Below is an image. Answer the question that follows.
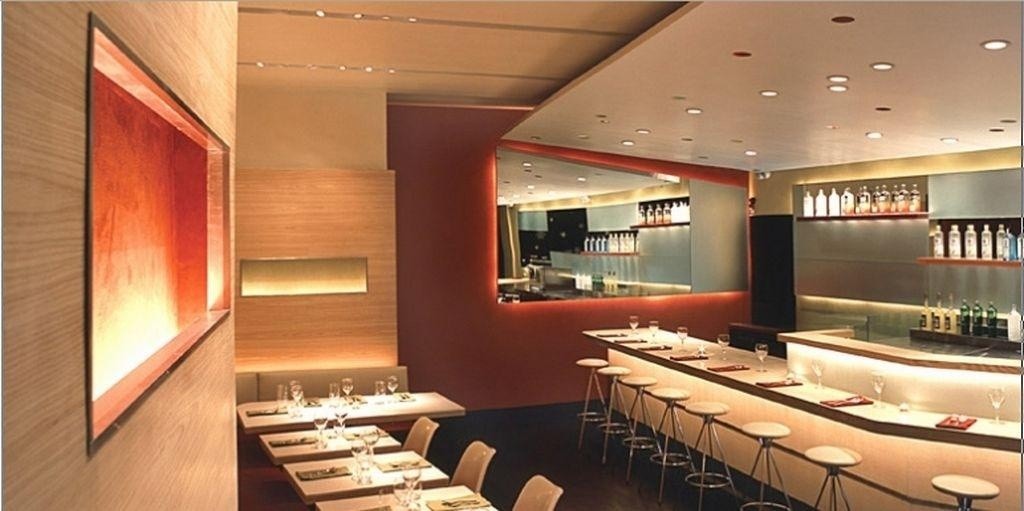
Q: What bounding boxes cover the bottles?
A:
[576,270,618,295]
[803,191,814,217]
[583,231,635,253]
[960,299,970,334]
[1008,304,1022,343]
[664,203,671,224]
[854,183,922,213]
[816,189,827,216]
[933,225,944,258]
[996,225,1005,260]
[828,187,840,216]
[647,204,655,225]
[920,295,932,331]
[671,202,690,222]
[656,204,663,224]
[964,225,977,260]
[944,294,957,334]
[932,292,944,333]
[981,224,993,259]
[987,301,997,337]
[972,301,982,335]
[840,188,855,215]
[639,206,646,225]
[948,225,961,258]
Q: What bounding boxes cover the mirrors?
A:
[494,146,751,306]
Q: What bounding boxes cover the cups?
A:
[360,426,380,466]
[342,377,354,397]
[649,321,659,344]
[334,405,349,435]
[402,461,422,492]
[411,482,422,508]
[717,334,730,361]
[628,315,639,335]
[359,466,372,485]
[811,358,825,392]
[313,411,328,441]
[290,385,304,408]
[986,384,1008,428]
[755,343,768,373]
[277,383,288,408]
[387,374,399,396]
[870,369,886,410]
[328,383,340,402]
[350,440,368,475]
[677,326,687,347]
[394,478,407,506]
[374,381,386,398]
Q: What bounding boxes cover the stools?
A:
[801,445,864,511]
[574,358,617,448]
[930,474,1000,511]
[649,386,698,501]
[595,365,638,466]
[619,375,664,483]
[682,401,737,511]
[737,420,794,511]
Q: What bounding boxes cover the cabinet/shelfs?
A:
[909,218,1023,352]
[796,213,930,223]
[776,327,1024,424]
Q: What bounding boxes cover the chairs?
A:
[402,414,443,460]
[509,474,564,511]
[451,439,499,496]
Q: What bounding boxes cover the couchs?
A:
[235,366,409,436]
